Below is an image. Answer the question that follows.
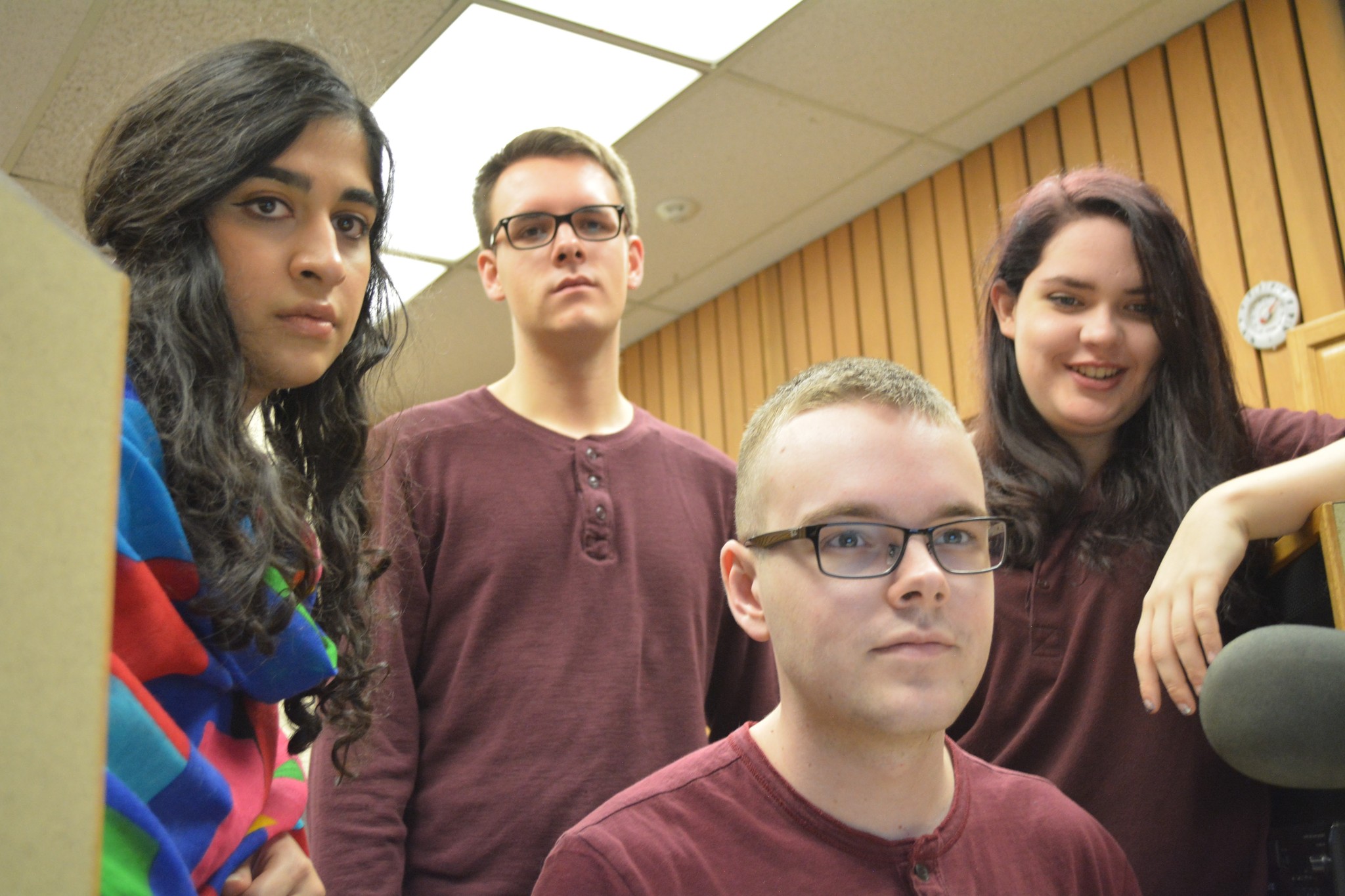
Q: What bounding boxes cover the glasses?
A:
[490,204,628,250]
[744,515,1010,579]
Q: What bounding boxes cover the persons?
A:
[312,127,786,896]
[79,36,409,896]
[963,169,1344,896]
[530,354,1144,896]
